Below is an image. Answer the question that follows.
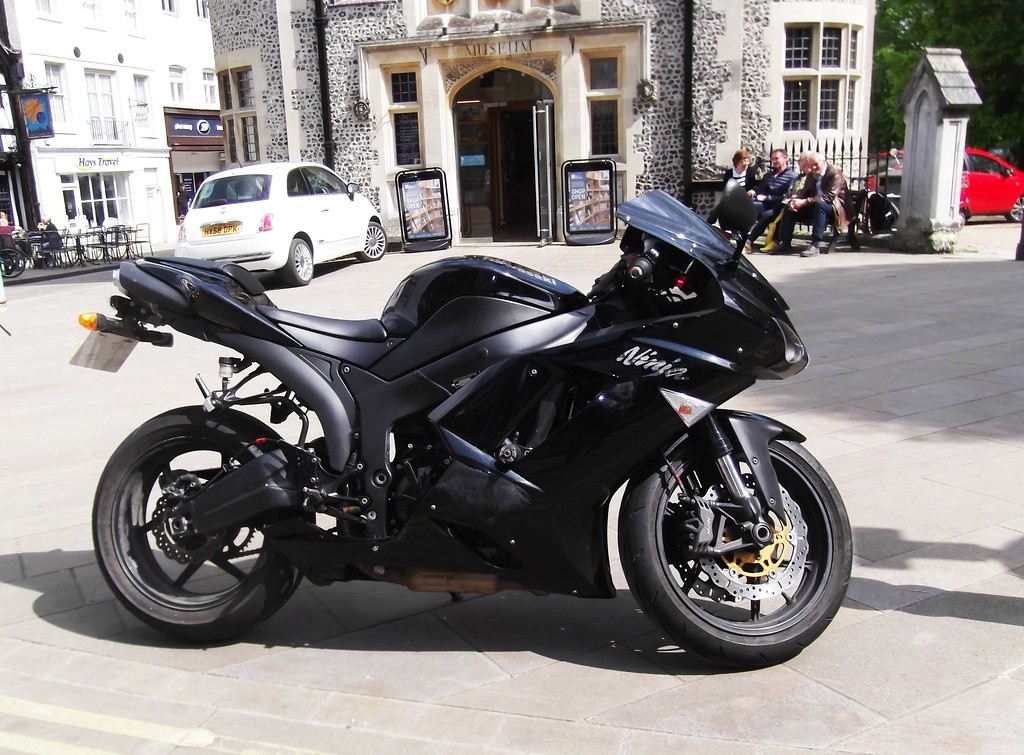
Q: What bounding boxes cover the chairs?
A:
[0,223,153,275]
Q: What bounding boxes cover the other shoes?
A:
[760,242,777,252]
[767,240,792,254]
[801,245,821,256]
[744,244,753,254]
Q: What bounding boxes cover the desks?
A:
[15,237,42,266]
[63,233,102,268]
[110,229,143,262]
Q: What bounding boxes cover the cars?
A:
[177,163,387,284]
[884,150,1024,227]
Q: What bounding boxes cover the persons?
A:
[769,152,858,258]
[36,215,64,269]
[722,149,811,254]
[0,209,10,226]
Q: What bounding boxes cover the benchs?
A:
[826,191,868,255]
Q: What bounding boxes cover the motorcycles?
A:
[74,189,854,672]
